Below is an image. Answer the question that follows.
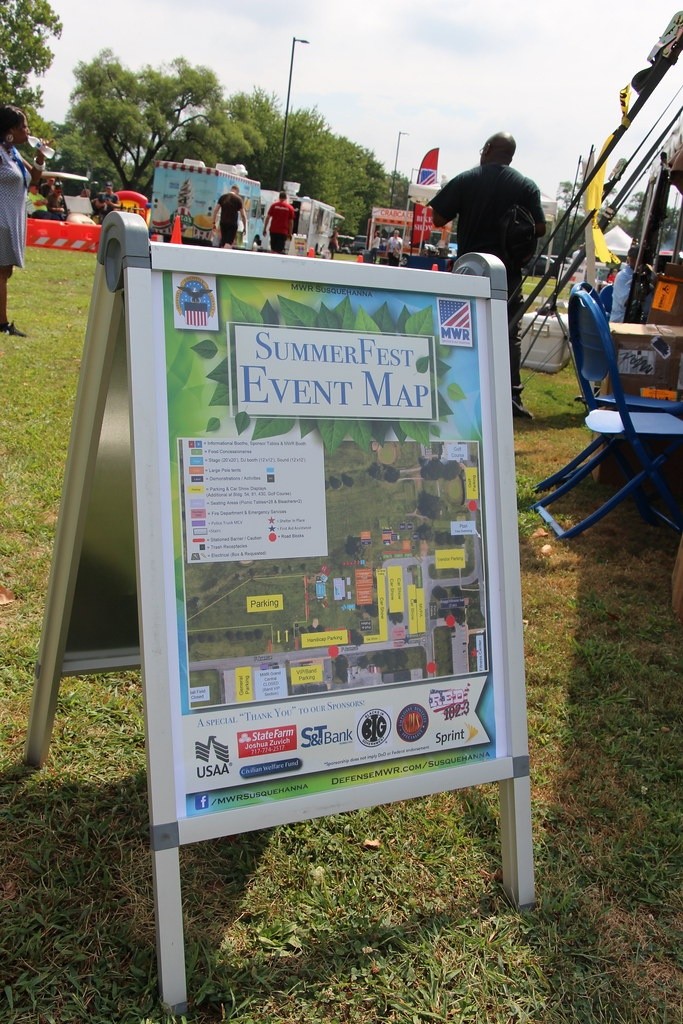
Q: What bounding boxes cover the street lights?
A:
[390,132,409,208]
[277,37,310,189]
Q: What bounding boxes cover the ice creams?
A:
[177,177,193,212]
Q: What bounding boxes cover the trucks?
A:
[265,190,345,257]
[148,158,264,252]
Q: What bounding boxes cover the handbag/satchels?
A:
[393,247,400,259]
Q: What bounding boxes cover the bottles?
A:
[27,135,55,159]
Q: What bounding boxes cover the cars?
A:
[521,255,584,279]
[398,243,455,272]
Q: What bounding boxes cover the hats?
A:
[106,181,112,188]
[628,247,639,259]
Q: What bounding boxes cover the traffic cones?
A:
[170,215,183,243]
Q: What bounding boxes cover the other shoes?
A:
[0,321,26,338]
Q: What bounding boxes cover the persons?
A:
[426,132,548,420]
[608,247,639,323]
[385,230,403,266]
[74,174,122,225]
[262,190,296,254]
[26,176,70,221]
[366,231,381,264]
[328,227,340,259]
[211,184,247,249]
[0,104,50,338]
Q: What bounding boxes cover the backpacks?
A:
[497,202,538,268]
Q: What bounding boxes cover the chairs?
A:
[527,281,683,537]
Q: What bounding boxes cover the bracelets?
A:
[34,156,46,171]
[212,222,215,224]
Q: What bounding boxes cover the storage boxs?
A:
[588,320,683,486]
[520,310,571,374]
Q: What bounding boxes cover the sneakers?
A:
[511,384,535,420]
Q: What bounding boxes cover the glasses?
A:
[479,146,485,156]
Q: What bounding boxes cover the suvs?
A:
[351,235,366,254]
